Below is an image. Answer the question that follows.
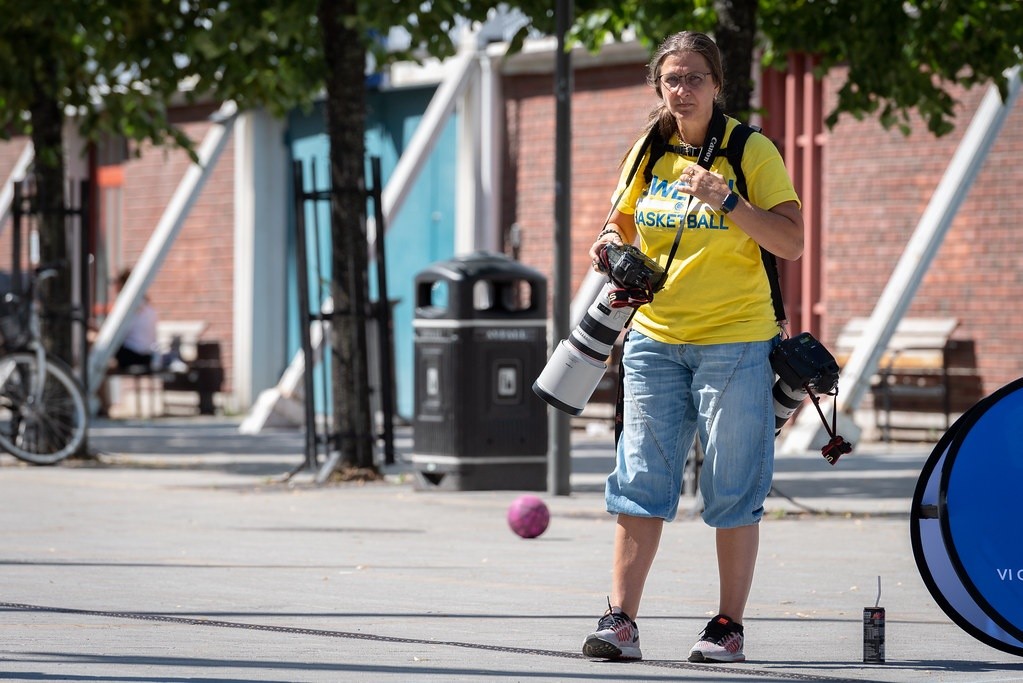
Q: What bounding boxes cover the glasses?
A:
[658,72,711,89]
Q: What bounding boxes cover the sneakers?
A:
[687,615,746,662]
[582,595,642,659]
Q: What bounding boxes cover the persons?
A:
[86,269,156,420]
[583,33,804,664]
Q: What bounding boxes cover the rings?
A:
[689,170,694,176]
[689,177,692,183]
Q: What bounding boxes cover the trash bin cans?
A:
[402,249,550,489]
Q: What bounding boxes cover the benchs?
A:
[117,317,212,417]
[824,316,961,447]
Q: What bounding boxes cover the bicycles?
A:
[0,292,88,466]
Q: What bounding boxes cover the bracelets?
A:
[597,229,623,243]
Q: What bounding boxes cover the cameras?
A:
[531,243,670,418]
[770,331,841,438]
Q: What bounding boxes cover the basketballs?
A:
[508,496,549,539]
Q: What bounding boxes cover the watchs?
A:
[712,191,739,216]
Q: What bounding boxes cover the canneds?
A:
[862,606,886,664]
